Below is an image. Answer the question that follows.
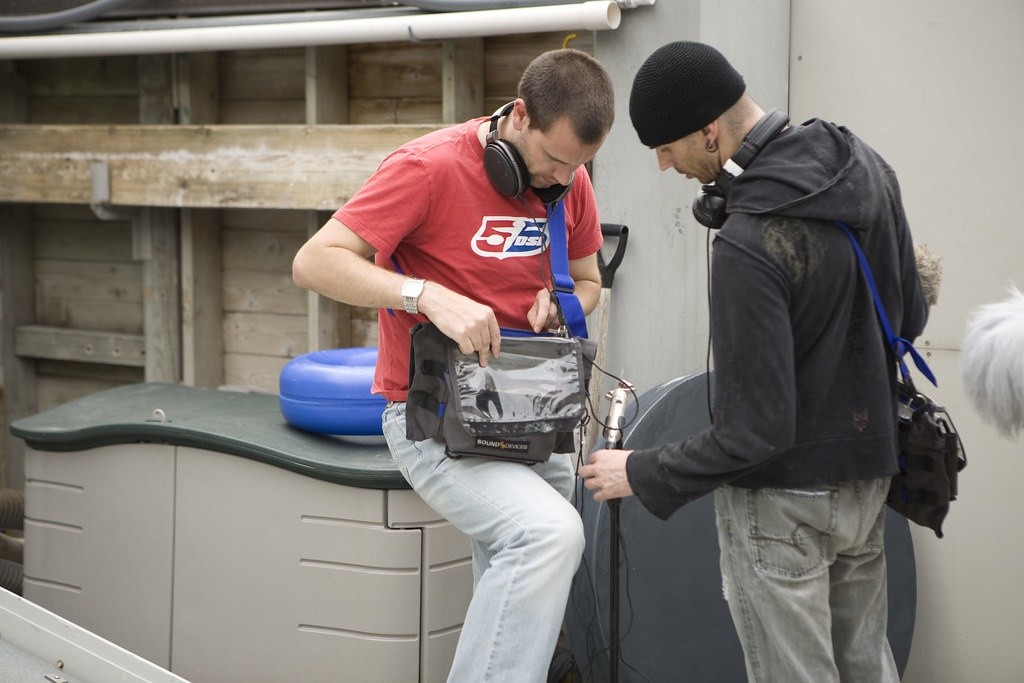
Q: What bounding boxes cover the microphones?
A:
[607,389,625,451]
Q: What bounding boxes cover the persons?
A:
[293,50,614,683]
[581,42,926,683]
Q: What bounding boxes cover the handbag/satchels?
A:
[406,322,598,464]
[885,383,966,538]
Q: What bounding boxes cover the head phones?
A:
[484,101,574,205]
[692,107,789,229]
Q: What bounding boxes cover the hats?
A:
[629,41,746,150]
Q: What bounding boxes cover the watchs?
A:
[402,276,426,314]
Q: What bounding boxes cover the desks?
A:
[6,381,474,683]
